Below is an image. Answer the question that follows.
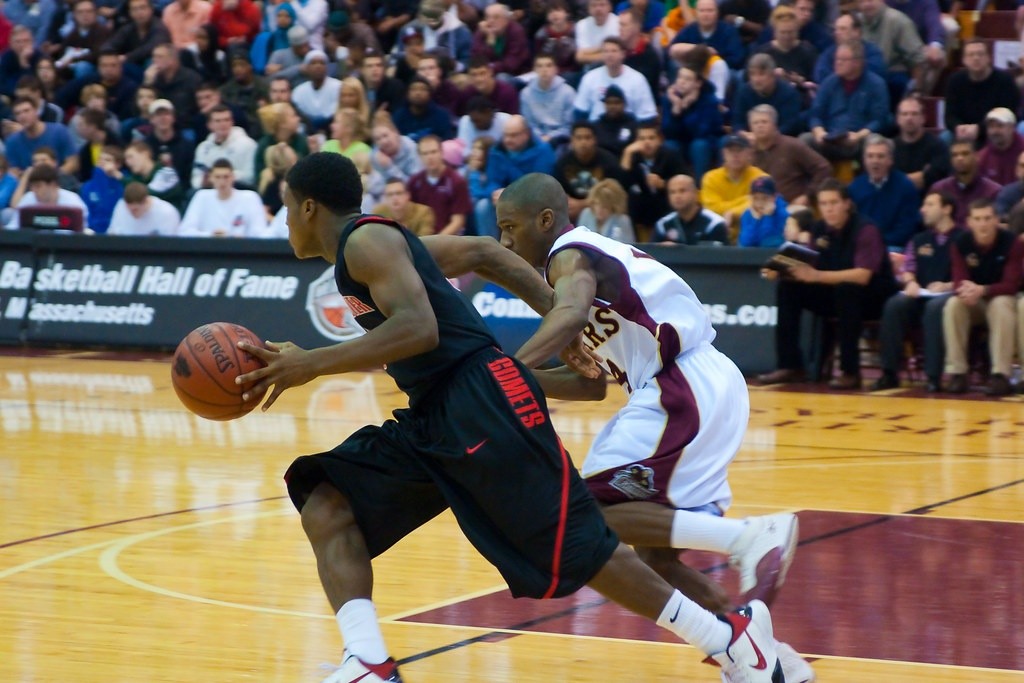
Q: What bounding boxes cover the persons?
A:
[496,172,798,616]
[0,0,1024,401]
[236,151,788,683]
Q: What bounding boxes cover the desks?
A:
[0,230,806,376]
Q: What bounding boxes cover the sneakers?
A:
[721,638,816,683]
[319,648,403,683]
[702,600,787,683]
[729,512,798,604]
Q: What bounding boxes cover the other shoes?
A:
[926,380,940,392]
[759,369,807,383]
[869,376,899,391]
[944,374,969,393]
[829,373,862,390]
[981,375,1013,397]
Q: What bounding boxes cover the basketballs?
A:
[170,321,268,421]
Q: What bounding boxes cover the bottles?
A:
[611,224,623,241]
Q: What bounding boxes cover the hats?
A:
[985,107,1016,124]
[149,98,173,114]
[720,135,752,148]
[751,178,776,194]
[287,26,310,45]
[606,84,624,101]
[401,26,424,43]
[230,47,251,66]
[327,11,350,30]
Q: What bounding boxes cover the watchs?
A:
[734,16,744,28]
